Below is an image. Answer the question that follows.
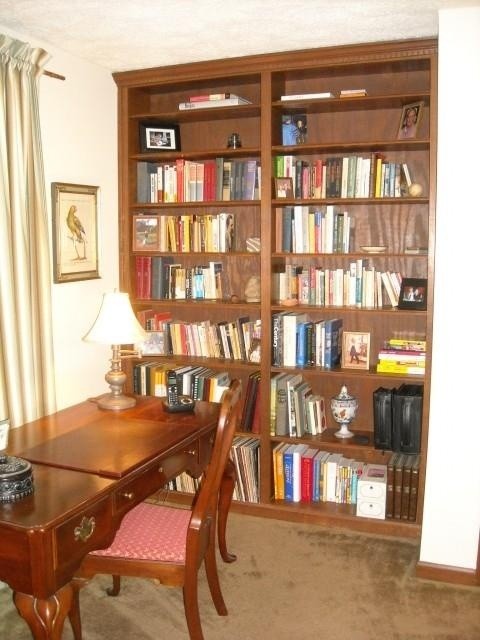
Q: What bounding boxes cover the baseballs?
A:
[408,184,424,197]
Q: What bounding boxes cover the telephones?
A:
[161,370,195,412]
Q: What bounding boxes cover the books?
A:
[338,92,369,98]
[273,311,426,374]
[134,359,261,434]
[136,157,262,203]
[372,383,423,454]
[164,438,259,503]
[273,442,421,521]
[178,98,248,113]
[340,88,370,94]
[137,308,261,359]
[279,258,404,306]
[161,213,234,253]
[190,91,254,105]
[280,91,336,101]
[277,154,413,197]
[135,255,223,299]
[275,205,356,253]
[270,373,327,437]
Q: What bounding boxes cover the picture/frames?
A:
[139,122,182,153]
[132,215,160,254]
[50,181,102,284]
[396,100,425,141]
[398,277,428,311]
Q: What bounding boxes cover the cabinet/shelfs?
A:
[111,34,438,541]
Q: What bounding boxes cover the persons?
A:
[408,287,423,302]
[297,119,307,135]
[282,115,300,146]
[399,108,417,138]
[144,226,149,245]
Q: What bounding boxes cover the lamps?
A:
[82,292,150,410]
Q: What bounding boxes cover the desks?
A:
[1,393,238,640]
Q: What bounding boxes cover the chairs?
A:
[70,378,243,640]
